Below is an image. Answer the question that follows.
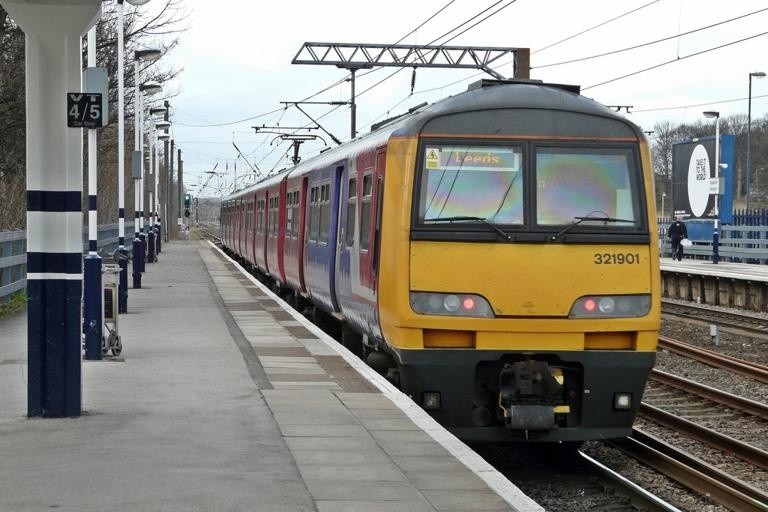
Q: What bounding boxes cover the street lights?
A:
[131,47,161,236]
[113,0,153,263]
[742,69,766,214]
[755,167,765,192]
[138,79,173,256]
[701,110,720,265]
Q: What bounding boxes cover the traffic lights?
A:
[184,193,191,210]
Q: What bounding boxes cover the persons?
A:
[667,214,688,261]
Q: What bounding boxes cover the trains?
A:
[219,75,668,463]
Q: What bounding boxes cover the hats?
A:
[677,215,682,222]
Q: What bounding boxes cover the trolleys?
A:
[82,261,125,359]
